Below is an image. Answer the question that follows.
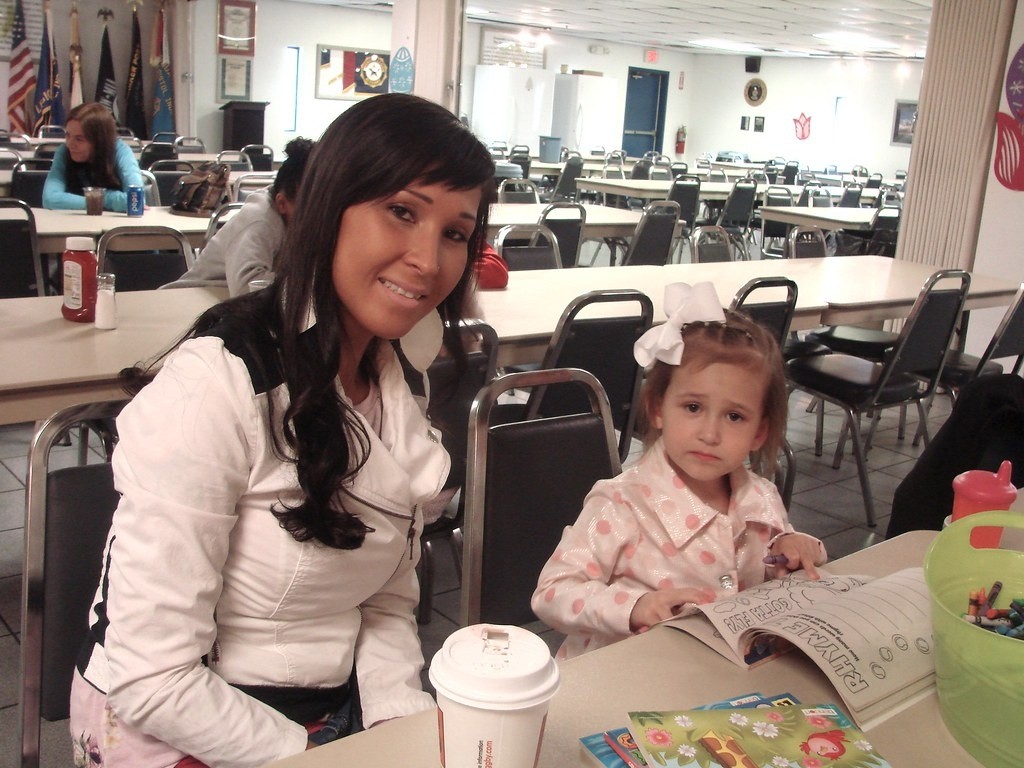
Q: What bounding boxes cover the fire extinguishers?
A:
[677,126,686,154]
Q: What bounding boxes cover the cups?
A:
[84,187,107,215]
[942,515,952,531]
[428,622,560,768]
[951,460,1018,549]
[248,279,274,293]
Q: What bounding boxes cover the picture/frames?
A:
[217,0,256,57]
[744,78,766,107]
[890,99,919,146]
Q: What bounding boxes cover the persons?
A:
[180,136,316,297]
[42,102,146,211]
[531,282,827,662]
[69,92,496,768]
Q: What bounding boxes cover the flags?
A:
[95,24,122,135]
[6,0,36,133]
[66,13,85,108]
[150,9,174,143]
[123,13,147,140]
[31,9,68,133]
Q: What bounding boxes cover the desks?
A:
[0,287,229,431]
[581,164,667,179]
[749,184,844,203]
[525,158,589,188]
[771,252,1024,343]
[0,127,1024,625]
[254,528,976,768]
[477,202,687,267]
[134,150,240,170]
[757,205,901,249]
[470,265,830,371]
[100,205,239,253]
[847,187,880,207]
[1,208,104,255]
[573,177,758,211]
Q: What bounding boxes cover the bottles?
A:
[95,273,118,330]
[61,236,98,322]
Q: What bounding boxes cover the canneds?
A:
[127,185,144,217]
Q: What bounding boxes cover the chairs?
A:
[16,394,136,768]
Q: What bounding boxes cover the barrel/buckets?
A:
[924,509,1023,767]
